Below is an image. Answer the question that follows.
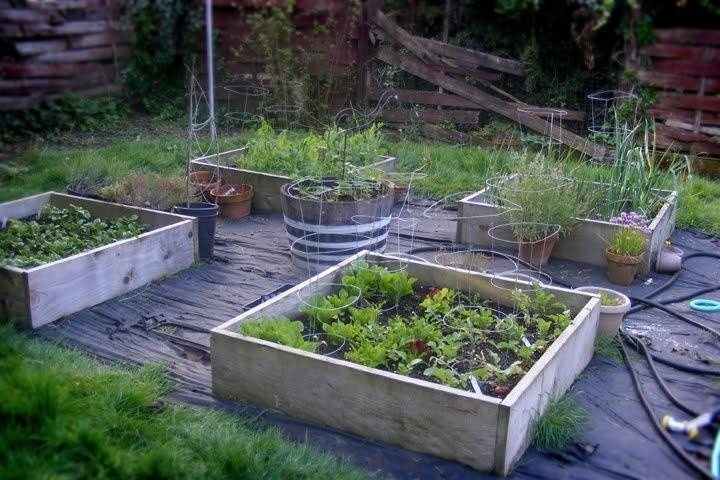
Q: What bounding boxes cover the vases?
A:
[601,245,644,286]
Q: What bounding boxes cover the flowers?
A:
[608,209,650,257]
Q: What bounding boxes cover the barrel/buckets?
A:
[174,201,219,259]
[280,177,394,272]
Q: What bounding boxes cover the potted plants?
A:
[280,114,395,272]
[452,96,679,276]
[1,190,198,329]
[209,256,611,479]
[188,110,396,214]
